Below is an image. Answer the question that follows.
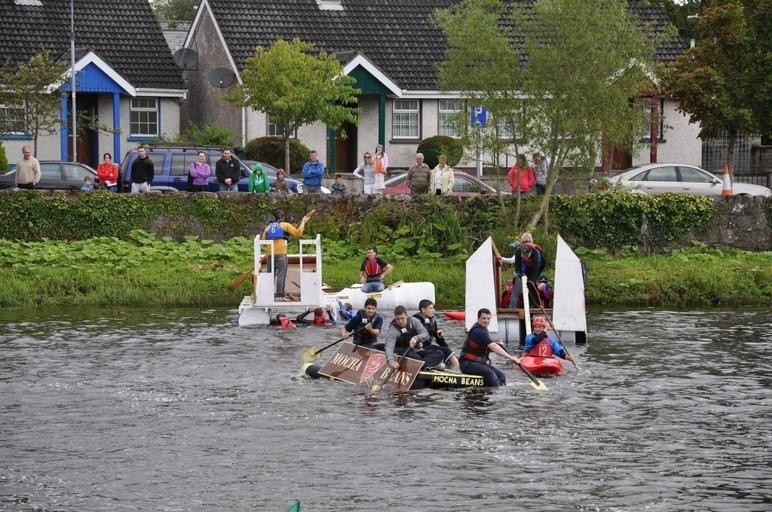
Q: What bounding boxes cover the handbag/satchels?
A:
[187,172,192,190]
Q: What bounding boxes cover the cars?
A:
[0,159,115,189]
[243,158,331,195]
[590,162,771,199]
[381,169,512,195]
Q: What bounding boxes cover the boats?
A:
[512,353,564,379]
[237,234,341,326]
[465,234,588,346]
[300,362,492,390]
[332,279,437,316]
[442,311,465,321]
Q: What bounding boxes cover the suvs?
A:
[117,143,304,192]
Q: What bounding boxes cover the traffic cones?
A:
[720,164,732,197]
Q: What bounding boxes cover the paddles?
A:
[228,211,314,292]
[498,341,547,390]
[303,323,373,362]
[526,281,584,374]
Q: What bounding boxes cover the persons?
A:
[98,153,119,192]
[330,175,347,194]
[431,155,454,195]
[302,150,324,193]
[341,298,385,350]
[248,163,269,193]
[459,308,521,387]
[81,176,93,191]
[337,296,357,321]
[353,152,377,193]
[508,155,536,196]
[412,299,459,368]
[537,275,553,308]
[530,152,551,195]
[15,145,41,190]
[406,153,431,194]
[270,313,296,329]
[385,305,445,370]
[189,151,211,192]
[216,148,240,192]
[373,145,388,188]
[262,207,310,296]
[502,279,523,308]
[271,169,288,193]
[361,247,395,292]
[131,147,154,194]
[525,317,573,361]
[296,305,336,325]
[497,234,542,307]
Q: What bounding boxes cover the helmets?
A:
[532,317,545,328]
